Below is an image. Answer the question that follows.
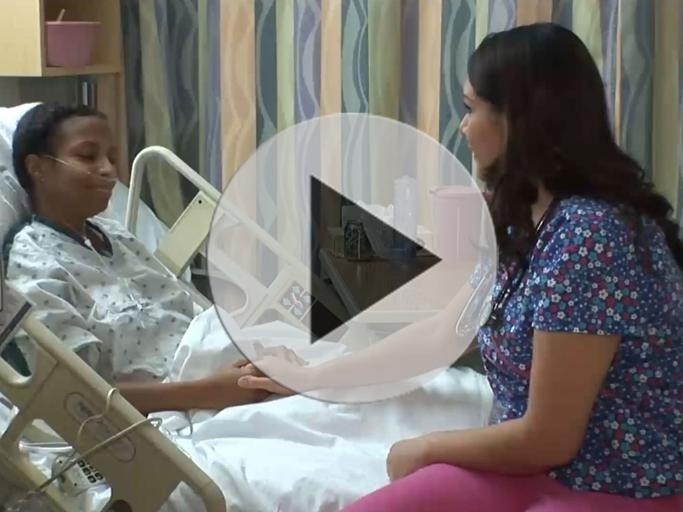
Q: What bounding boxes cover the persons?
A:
[5,101,270,412]
[233,23,683,512]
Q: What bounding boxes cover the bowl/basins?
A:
[42,19,103,68]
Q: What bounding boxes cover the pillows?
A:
[0,102,45,251]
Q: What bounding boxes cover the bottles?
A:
[393,171,419,264]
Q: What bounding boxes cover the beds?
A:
[0,101,494,512]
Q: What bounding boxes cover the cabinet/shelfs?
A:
[0,0,129,184]
